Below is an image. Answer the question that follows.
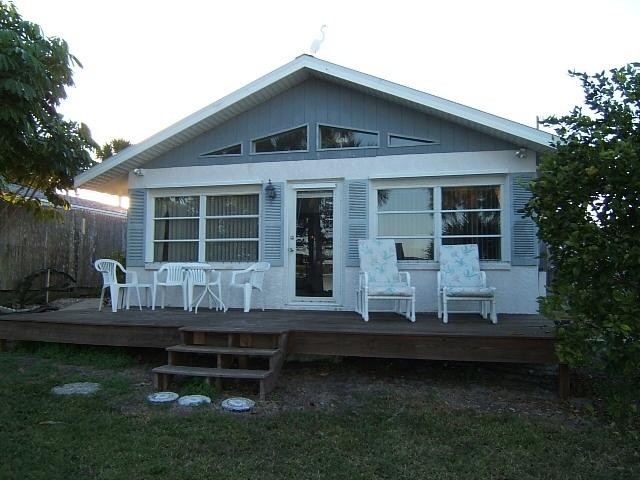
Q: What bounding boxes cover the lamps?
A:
[263,178,277,199]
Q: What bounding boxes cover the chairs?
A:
[355,237,498,325]
[94,257,273,314]
